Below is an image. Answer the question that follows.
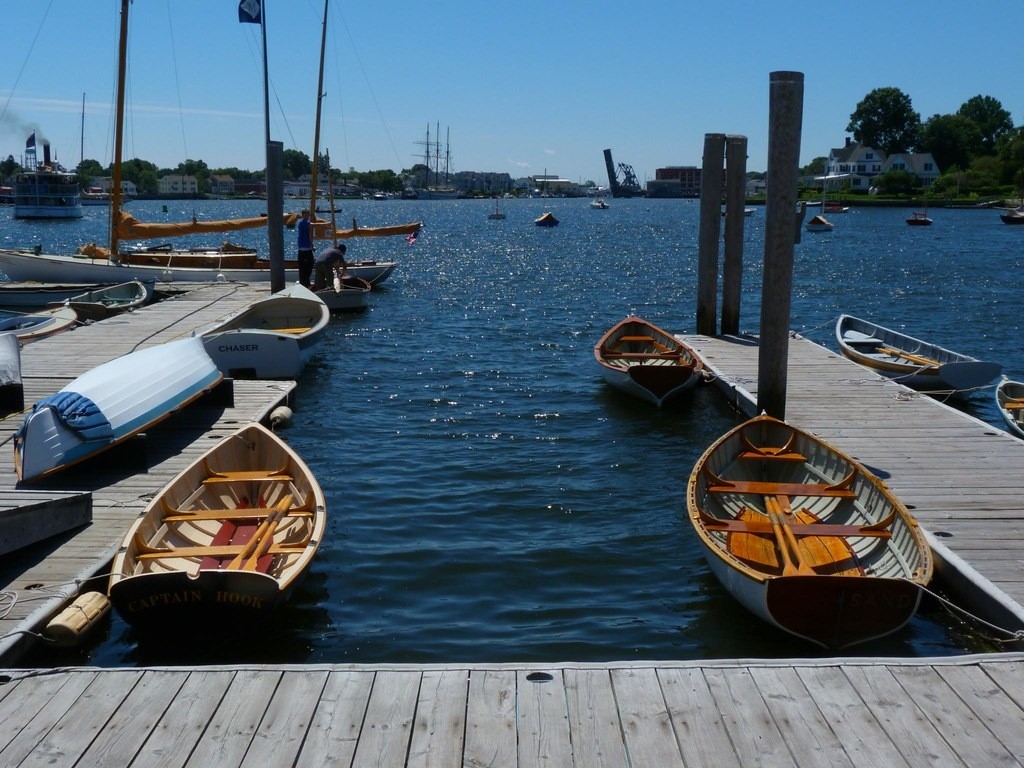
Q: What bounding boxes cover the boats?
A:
[804,174,834,232]
[487,207,506,219]
[0,280,219,312]
[417,121,458,200]
[65,278,147,320]
[999,212,1024,225]
[994,376,1024,435]
[819,207,850,213]
[533,212,560,226]
[744,208,757,216]
[593,313,705,409]
[0,305,78,345]
[800,200,840,207]
[197,281,332,380]
[12,333,224,483]
[905,175,933,225]
[835,313,1005,401]
[312,274,371,309]
[10,138,86,221]
[120,240,259,270]
[0,186,115,205]
[104,420,327,639]
[0,0,398,300]
[589,200,610,209]
[685,412,935,654]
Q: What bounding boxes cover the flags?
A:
[25,133,35,149]
[237,0,262,23]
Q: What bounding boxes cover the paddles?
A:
[224,491,296,572]
[874,346,942,366]
[762,492,817,576]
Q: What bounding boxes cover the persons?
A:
[297,209,316,292]
[315,243,349,290]
